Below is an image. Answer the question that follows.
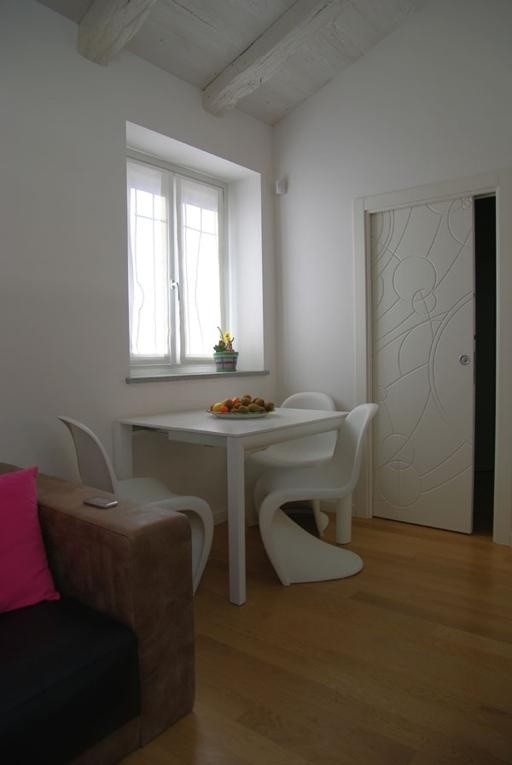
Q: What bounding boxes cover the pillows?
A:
[1,467,61,612]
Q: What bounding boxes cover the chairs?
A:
[248,392,339,538]
[56,415,214,598]
[0,462,194,764]
[253,403,380,586]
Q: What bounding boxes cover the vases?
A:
[214,352,238,372]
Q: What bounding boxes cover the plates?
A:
[207,409,276,419]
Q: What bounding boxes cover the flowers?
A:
[212,328,235,352]
